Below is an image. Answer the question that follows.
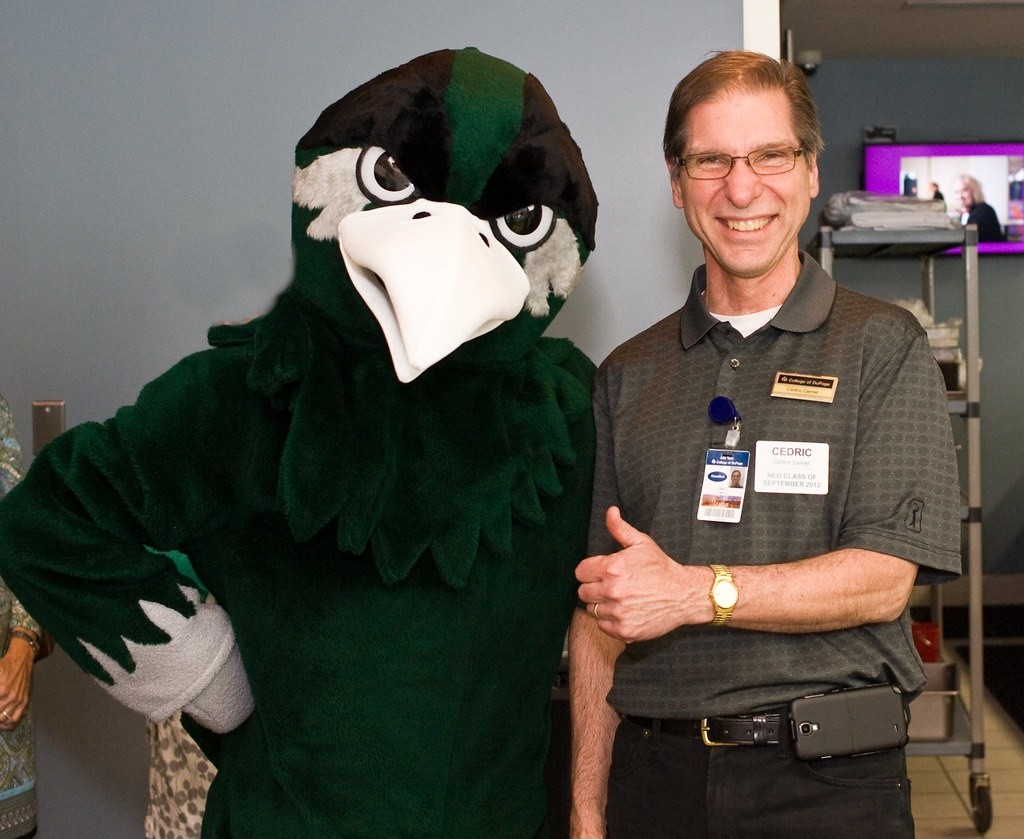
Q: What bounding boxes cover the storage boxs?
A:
[907,660,962,743]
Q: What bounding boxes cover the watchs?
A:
[708,564,738,627]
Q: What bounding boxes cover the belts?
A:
[622,711,796,749]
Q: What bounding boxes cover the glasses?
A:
[676,144,806,179]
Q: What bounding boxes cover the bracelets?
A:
[10,630,41,655]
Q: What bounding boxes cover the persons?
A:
[955,174,1007,244]
[0,395,52,839]
[929,182,944,200]
[568,50,962,839]
[730,470,744,488]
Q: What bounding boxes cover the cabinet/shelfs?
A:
[806,214,1008,777]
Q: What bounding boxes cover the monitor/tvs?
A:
[859,140,1024,255]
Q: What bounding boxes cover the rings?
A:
[593,603,602,620]
[3,711,10,719]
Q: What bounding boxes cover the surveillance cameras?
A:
[795,50,822,76]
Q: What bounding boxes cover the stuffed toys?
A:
[0,50,606,839]
